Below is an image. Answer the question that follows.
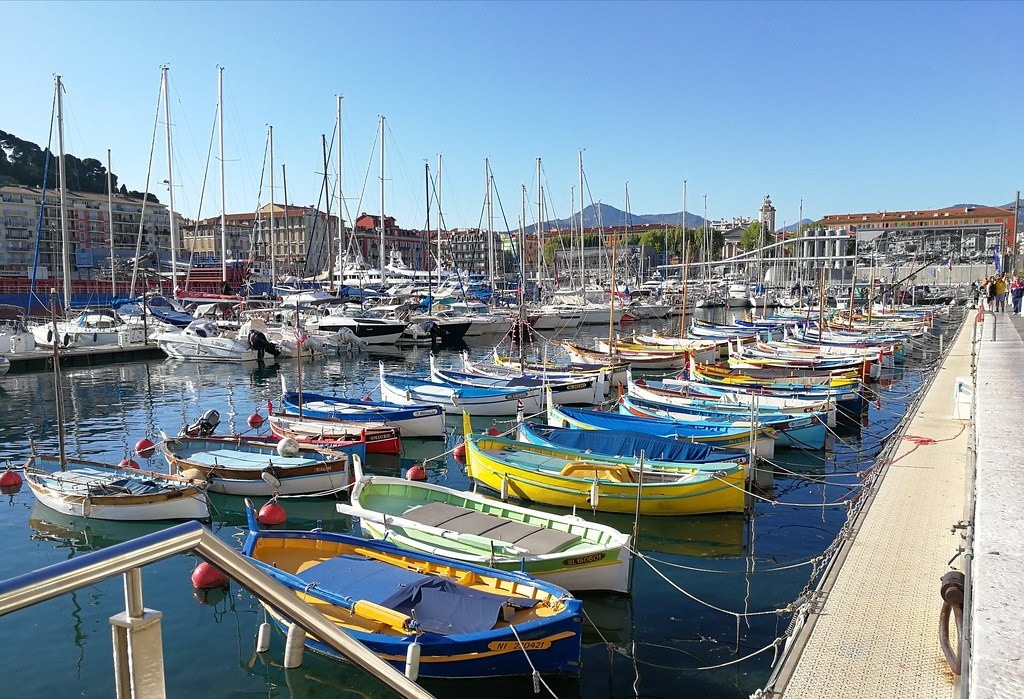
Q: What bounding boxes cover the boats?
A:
[25,288,211,521]
[451,393,757,516]
[336,448,645,597]
[242,498,584,678]
[156,409,349,503]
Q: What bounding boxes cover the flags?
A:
[948,260,952,271]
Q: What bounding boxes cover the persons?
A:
[969,274,1010,313]
[1011,276,1024,315]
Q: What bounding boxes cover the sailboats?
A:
[0,45,954,452]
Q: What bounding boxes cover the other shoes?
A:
[994,309,999,312]
[1017,312,1021,315]
[1012,312,1016,315]
[1002,309,1004,312]
